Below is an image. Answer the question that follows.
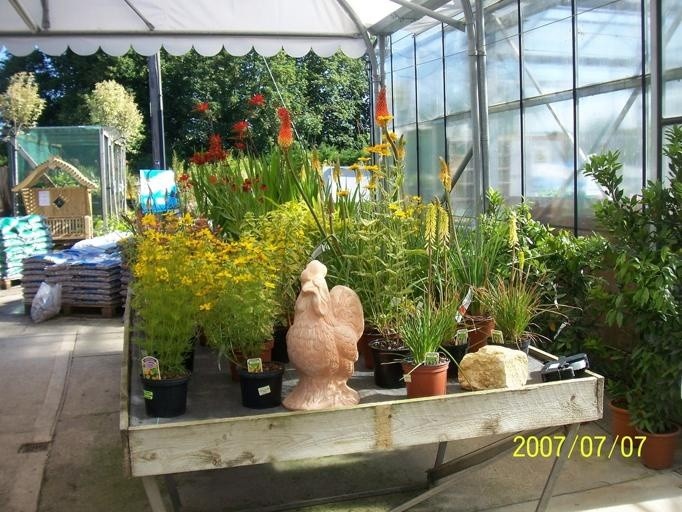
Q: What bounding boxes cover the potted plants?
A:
[579,121,682,470]
[129,200,313,420]
[338,190,584,399]
